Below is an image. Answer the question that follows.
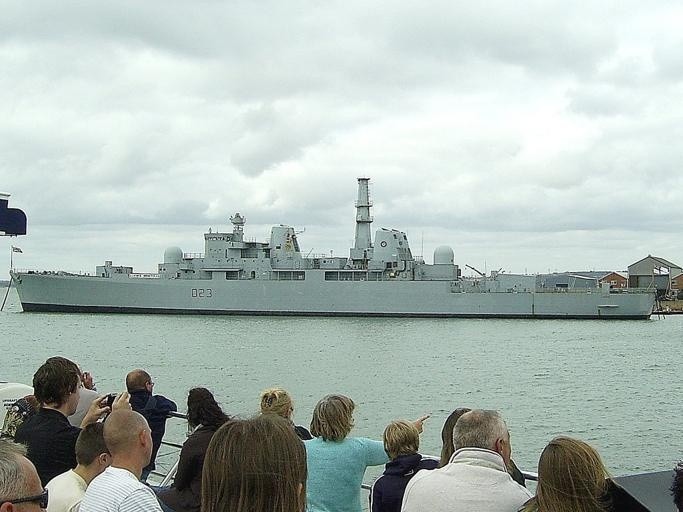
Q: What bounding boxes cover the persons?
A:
[1,358,132,488]
[126,368,176,480]
[300,393,430,510]
[517,436,609,511]
[151,388,232,511]
[1,439,49,510]
[669,459,683,512]
[202,414,307,510]
[402,409,533,509]
[259,388,312,439]
[79,409,163,511]
[43,422,112,510]
[370,420,440,510]
[439,407,526,488]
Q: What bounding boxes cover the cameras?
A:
[106,392,122,407]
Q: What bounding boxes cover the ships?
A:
[8,175,658,321]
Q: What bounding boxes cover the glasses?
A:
[0,488,49,510]
